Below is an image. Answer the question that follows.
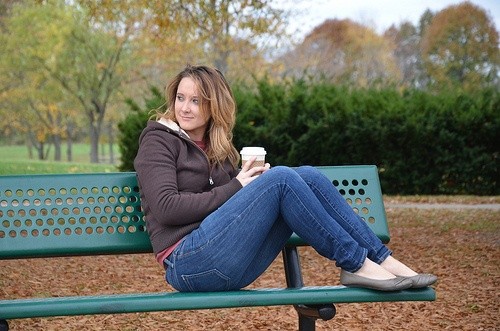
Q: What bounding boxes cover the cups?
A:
[240,147,267,176]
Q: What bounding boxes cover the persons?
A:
[134,65,438,293]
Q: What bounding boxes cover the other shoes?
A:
[395,273,439,289]
[339,269,412,291]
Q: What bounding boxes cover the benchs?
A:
[0,165,436,331]
